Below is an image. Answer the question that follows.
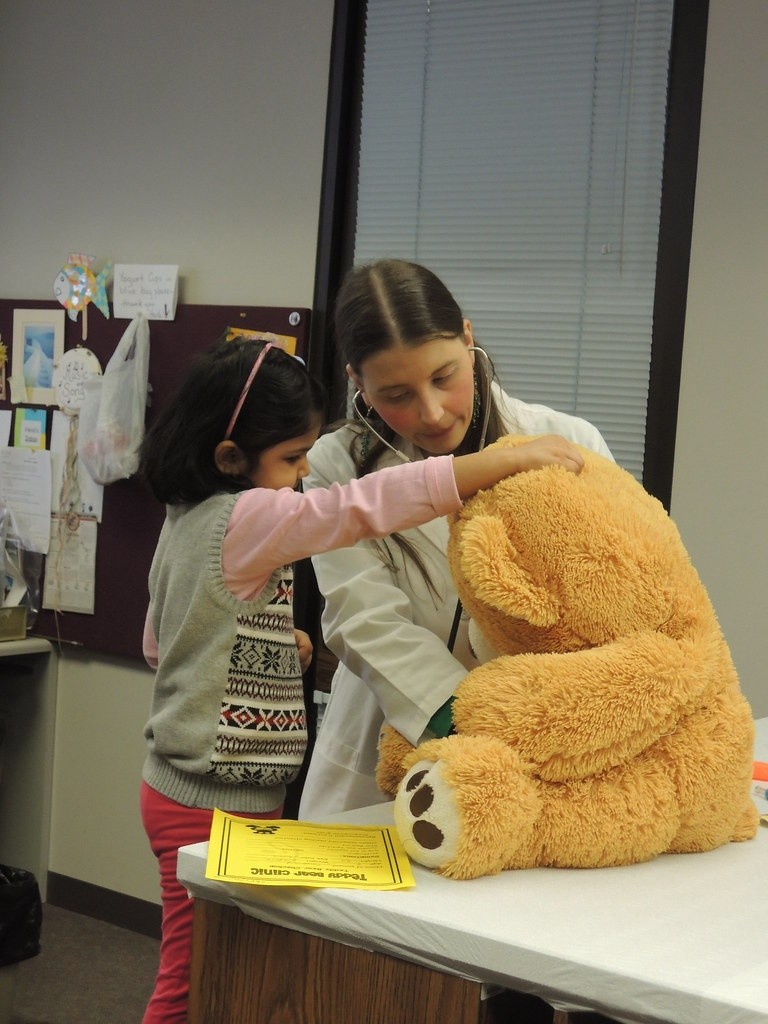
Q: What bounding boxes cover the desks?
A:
[176,717,768,1024]
[0,636,58,904]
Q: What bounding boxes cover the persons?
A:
[298,256,617,824]
[135,337,586,1024]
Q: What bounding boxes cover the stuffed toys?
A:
[374,435,760,881]
